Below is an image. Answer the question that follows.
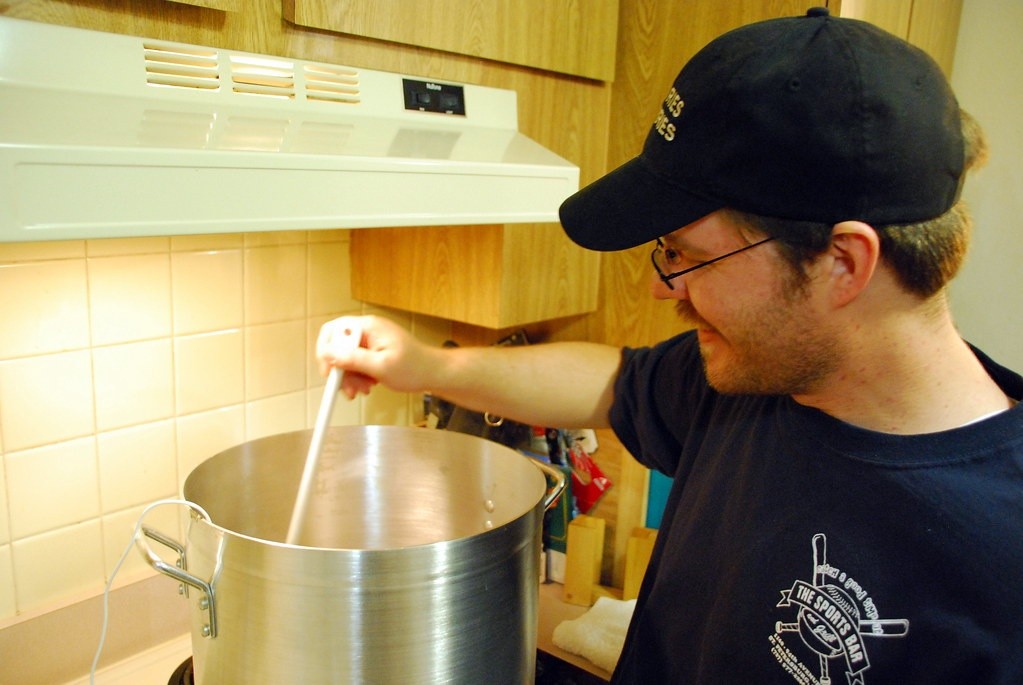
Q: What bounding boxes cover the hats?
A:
[559,7,966,251]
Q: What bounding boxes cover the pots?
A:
[134,425,567,684]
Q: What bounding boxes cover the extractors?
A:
[1,16,580,242]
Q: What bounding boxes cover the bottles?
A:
[530,424,551,476]
[540,541,548,586]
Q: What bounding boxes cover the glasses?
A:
[651,231,789,290]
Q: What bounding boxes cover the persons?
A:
[315,7,1023,685]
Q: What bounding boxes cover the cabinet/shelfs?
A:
[1,0,964,587]
